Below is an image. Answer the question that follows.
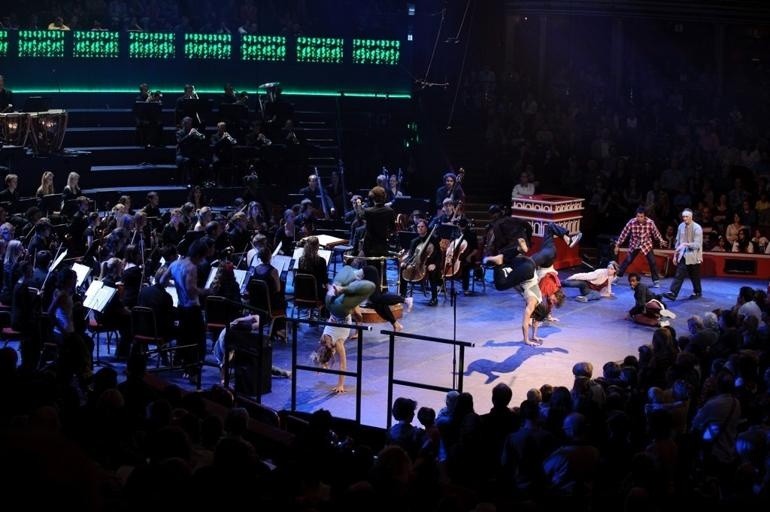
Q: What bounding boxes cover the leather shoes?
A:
[428,297,438,306]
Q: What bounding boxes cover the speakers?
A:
[235,333,272,397]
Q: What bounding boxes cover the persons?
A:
[47,17,71,32]
[0,73,769,512]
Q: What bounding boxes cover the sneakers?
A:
[653,280,660,288]
[657,309,677,327]
[395,297,414,332]
[663,293,677,301]
[568,233,582,248]
[576,296,588,302]
[688,292,702,300]
[612,278,619,285]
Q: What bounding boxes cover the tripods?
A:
[93,312,114,367]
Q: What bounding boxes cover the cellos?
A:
[441,219,475,278]
[401,224,438,282]
